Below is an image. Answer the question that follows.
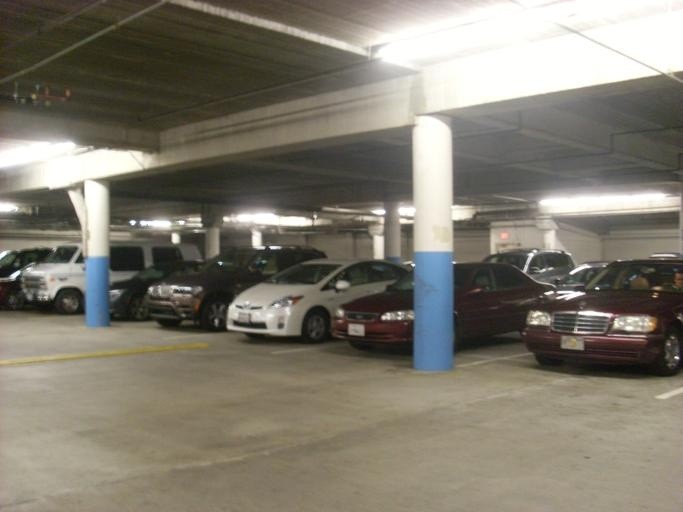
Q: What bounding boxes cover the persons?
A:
[662,272,683,293]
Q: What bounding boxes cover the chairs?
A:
[629,274,652,289]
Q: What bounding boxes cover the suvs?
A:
[136,242,332,332]
[0,245,53,275]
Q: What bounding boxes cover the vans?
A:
[21,241,203,317]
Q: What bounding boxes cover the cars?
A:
[329,257,562,358]
[109,257,205,325]
[518,253,682,376]
[0,260,42,310]
[221,256,409,347]
[399,248,618,290]
